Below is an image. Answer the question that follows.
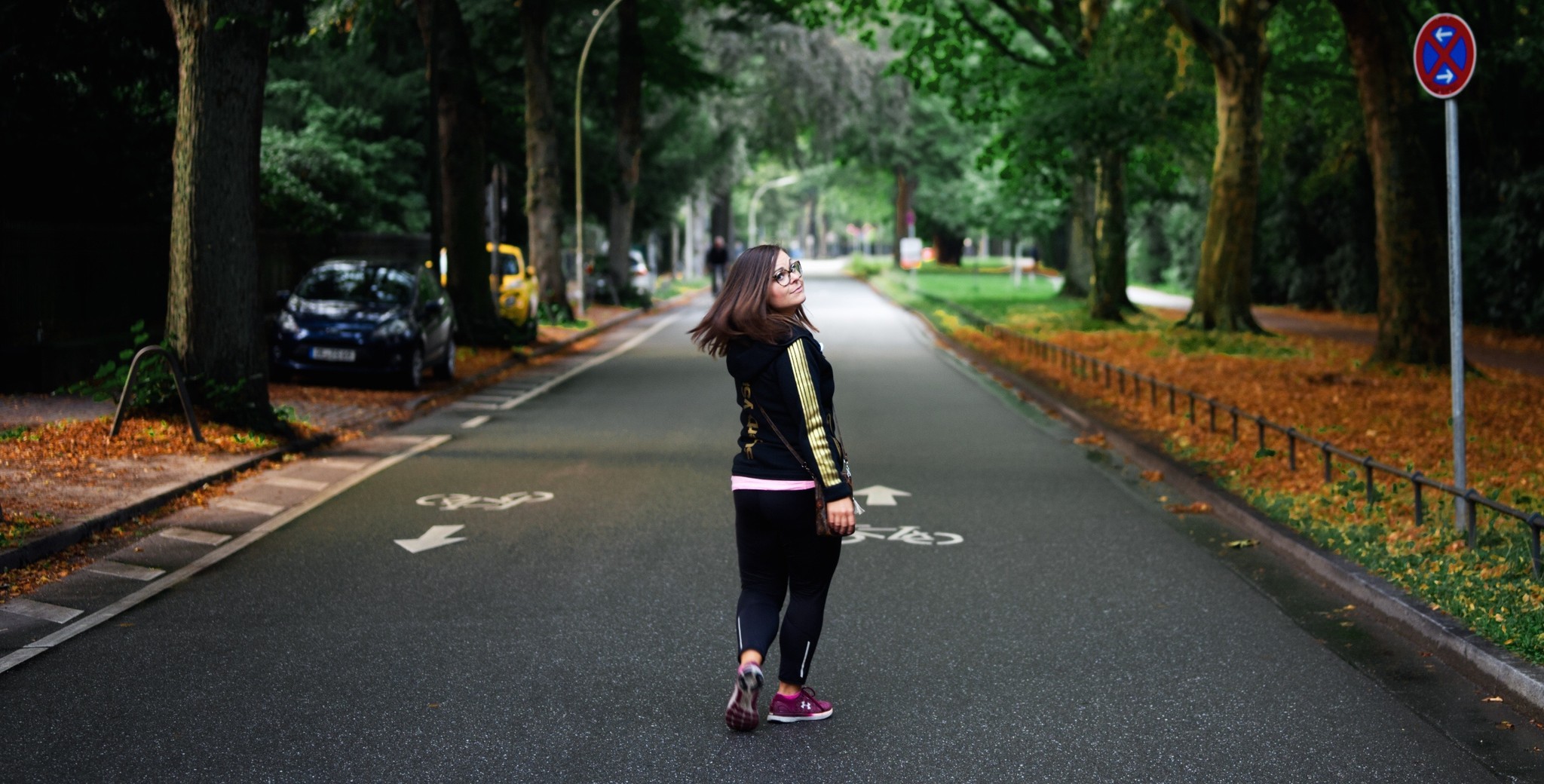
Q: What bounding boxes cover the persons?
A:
[686,246,856,731]
[705,236,745,297]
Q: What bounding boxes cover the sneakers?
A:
[726,661,764,731]
[768,686,833,723]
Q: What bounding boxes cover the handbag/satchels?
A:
[813,472,865,536]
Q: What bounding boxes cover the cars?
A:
[558,219,656,314]
[424,241,542,353]
[270,257,460,395]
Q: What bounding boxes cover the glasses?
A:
[772,260,802,286]
